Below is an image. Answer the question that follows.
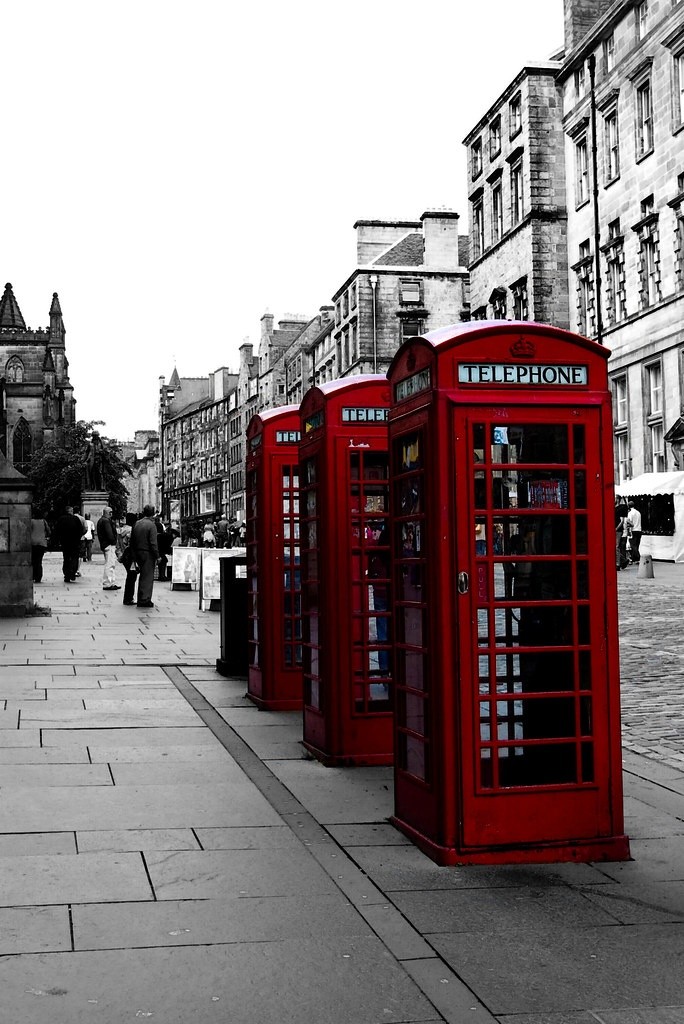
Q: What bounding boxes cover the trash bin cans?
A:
[217,552,254,677]
[283,553,302,663]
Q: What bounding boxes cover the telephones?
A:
[505,518,559,601]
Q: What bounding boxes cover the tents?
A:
[614,471,684,565]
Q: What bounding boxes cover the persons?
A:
[70,505,88,577]
[614,493,642,572]
[80,512,95,562]
[357,513,525,559]
[56,505,83,582]
[31,509,52,583]
[80,430,110,492]
[120,532,174,605]
[368,525,401,691]
[114,500,247,578]
[129,504,159,607]
[97,507,122,591]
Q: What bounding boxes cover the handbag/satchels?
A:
[43,519,50,541]
[122,546,140,574]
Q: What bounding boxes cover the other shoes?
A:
[103,585,121,590]
[75,572,82,577]
[127,601,137,605]
